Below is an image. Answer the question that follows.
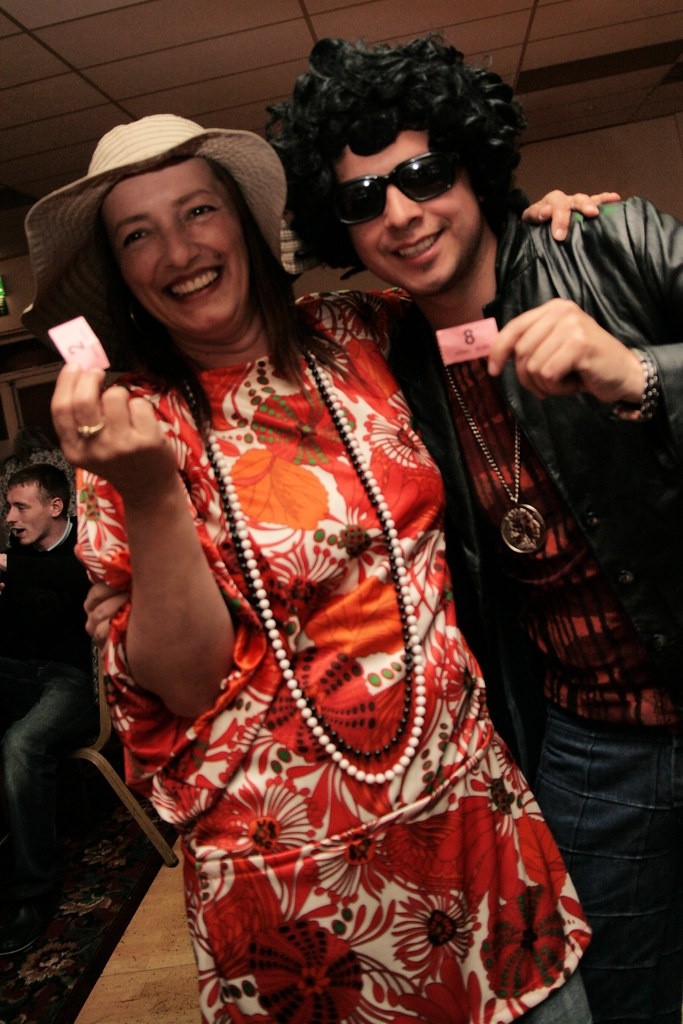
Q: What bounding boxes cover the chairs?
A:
[66,642,180,867]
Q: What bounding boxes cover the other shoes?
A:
[0,895,58,958]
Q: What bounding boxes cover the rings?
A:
[74,418,102,439]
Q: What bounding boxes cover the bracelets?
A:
[610,347,659,423]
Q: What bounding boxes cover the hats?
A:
[19,111,294,351]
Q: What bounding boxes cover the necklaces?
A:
[181,342,427,786]
[444,363,546,557]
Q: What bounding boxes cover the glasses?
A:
[327,149,462,226]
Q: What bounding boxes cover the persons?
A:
[82,35,683,1023]
[0,425,94,959]
[15,110,620,1024]
